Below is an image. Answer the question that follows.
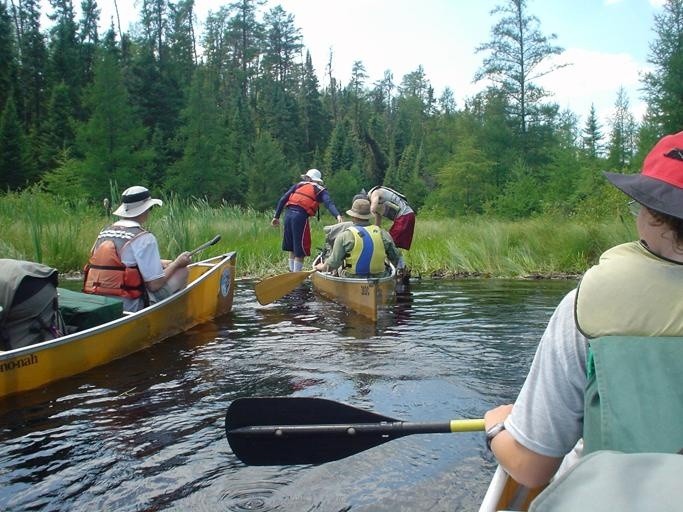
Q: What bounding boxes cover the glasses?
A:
[626,198,640,215]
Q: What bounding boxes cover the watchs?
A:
[483,418,509,452]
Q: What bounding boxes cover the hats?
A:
[301,169,325,184]
[111,185,162,217]
[347,189,375,220]
[603,129,683,218]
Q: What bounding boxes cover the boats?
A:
[310,246,399,321]
[1,243,240,402]
[472,430,587,512]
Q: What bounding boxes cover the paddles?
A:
[225,395,487,466]
[254,269,317,306]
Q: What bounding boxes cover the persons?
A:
[77,184,193,313]
[319,189,372,265]
[350,184,416,250]
[269,168,346,274]
[477,129,683,510]
[310,198,405,281]
